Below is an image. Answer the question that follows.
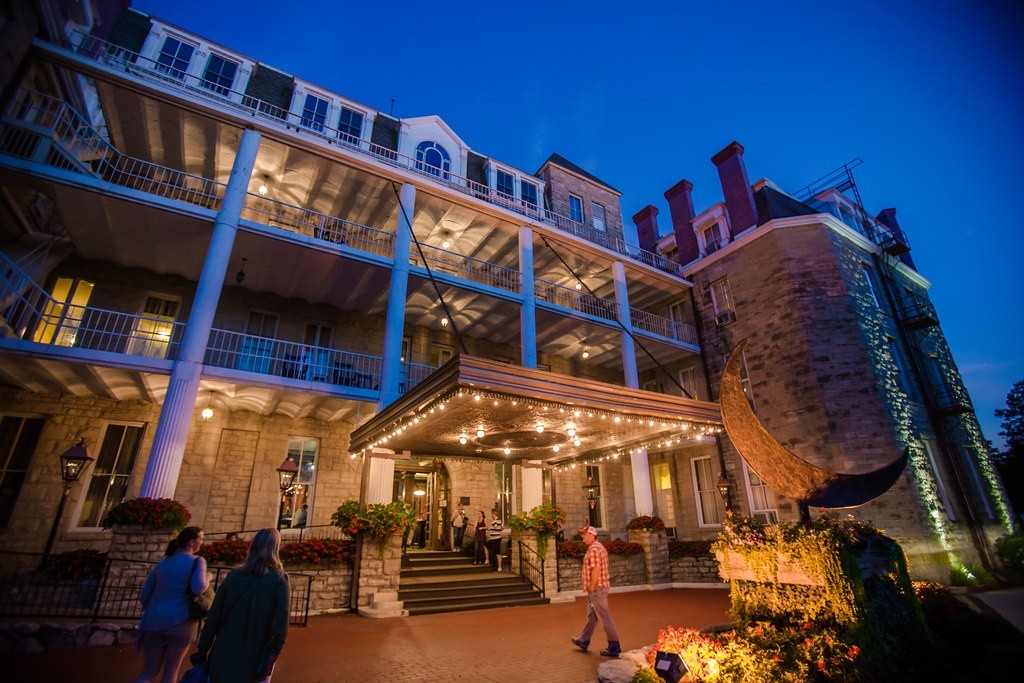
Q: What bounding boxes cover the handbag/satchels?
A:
[186,557,216,618]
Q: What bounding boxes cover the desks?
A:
[354,372,373,390]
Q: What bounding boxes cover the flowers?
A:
[624,513,670,533]
[99,497,193,534]
[510,503,568,535]
[328,499,420,542]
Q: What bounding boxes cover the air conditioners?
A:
[753,508,775,527]
[717,310,735,326]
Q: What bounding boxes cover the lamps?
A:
[536,424,544,434]
[459,437,468,445]
[259,173,271,196]
[442,231,450,250]
[413,484,425,495]
[582,339,589,359]
[441,312,449,328]
[202,390,216,420]
[566,428,576,436]
[236,258,248,284]
[654,650,689,683]
[477,429,485,438]
[576,275,582,290]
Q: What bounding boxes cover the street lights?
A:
[716,473,732,523]
[29,436,95,588]
[275,457,298,533]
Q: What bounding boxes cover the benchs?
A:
[282,353,356,387]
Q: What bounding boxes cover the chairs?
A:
[303,350,330,384]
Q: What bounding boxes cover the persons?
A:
[451,502,467,552]
[292,504,308,528]
[472,508,504,573]
[181,527,292,683]
[570,525,622,657]
[132,525,217,683]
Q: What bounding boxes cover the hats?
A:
[578,526,598,537]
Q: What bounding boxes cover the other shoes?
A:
[484,560,490,564]
[599,649,621,656]
[497,568,503,573]
[571,637,590,651]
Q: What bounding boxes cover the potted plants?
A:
[948,563,993,593]
